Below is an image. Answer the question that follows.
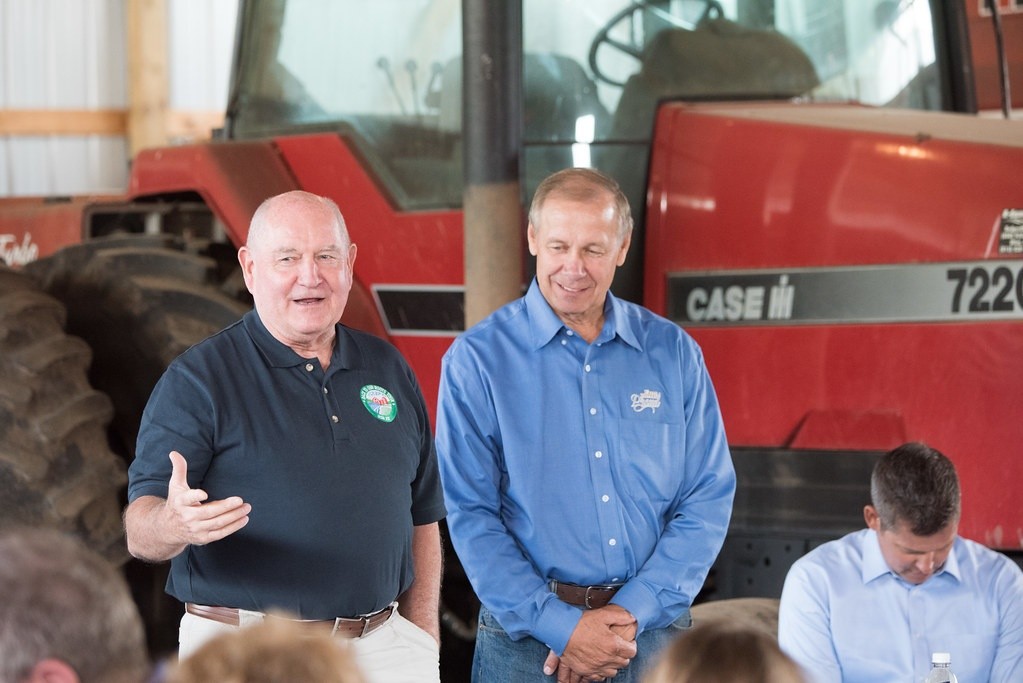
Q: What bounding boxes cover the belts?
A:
[187,603,394,640]
[547,581,618,610]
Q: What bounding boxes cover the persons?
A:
[124,189,448,682]
[779,442,1023,683]
[0,529,162,683]
[646,617,807,683]
[166,617,364,683]
[432,168,737,683]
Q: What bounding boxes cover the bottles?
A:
[924,654,957,683]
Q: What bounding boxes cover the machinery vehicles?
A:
[0,0,1022,648]
